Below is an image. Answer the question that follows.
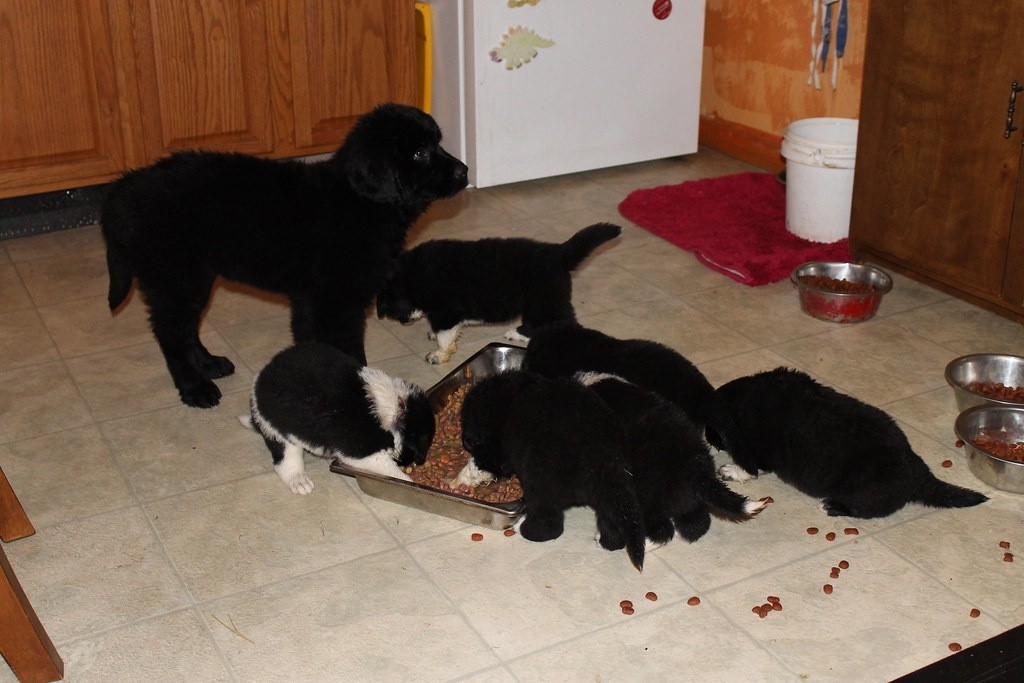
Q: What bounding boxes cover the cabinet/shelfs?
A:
[1,2,427,199]
[849,0,1024,324]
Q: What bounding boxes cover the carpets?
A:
[618,172,854,288]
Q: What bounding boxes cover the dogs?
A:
[375,222,621,366]
[98,102,469,408]
[235,341,452,496]
[450,323,990,574]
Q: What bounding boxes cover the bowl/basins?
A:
[944,353,1024,414]
[954,404,1024,494]
[791,261,893,323]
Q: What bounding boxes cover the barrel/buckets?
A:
[780,117,859,242]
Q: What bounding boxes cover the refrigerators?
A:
[431,0,705,189]
